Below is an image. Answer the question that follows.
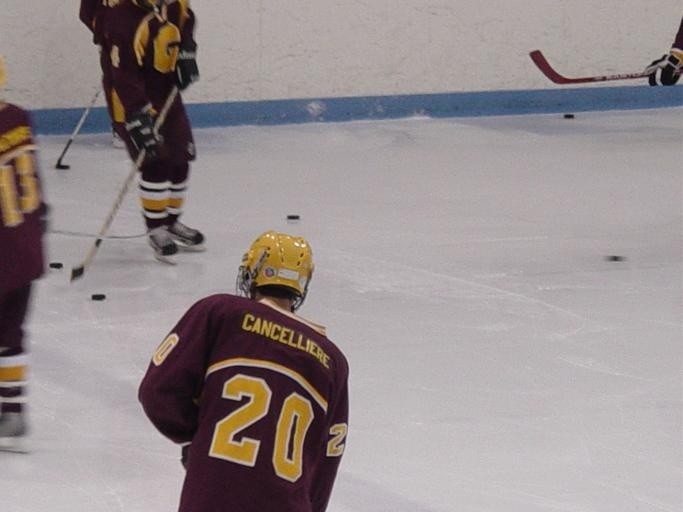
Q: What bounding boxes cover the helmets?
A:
[238,230,313,300]
[0,54,9,88]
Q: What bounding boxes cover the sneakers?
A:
[0,412,26,437]
[166,219,204,246]
[145,226,181,257]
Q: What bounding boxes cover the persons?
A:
[137,230,353,512]
[1,55,49,442]
[647,21,683,87]
[75,1,206,263]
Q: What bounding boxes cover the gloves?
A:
[642,47,683,86]
[123,111,162,161]
[174,53,200,91]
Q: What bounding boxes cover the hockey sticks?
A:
[55,86,102,169]
[529,50,653,83]
[69,84,178,281]
